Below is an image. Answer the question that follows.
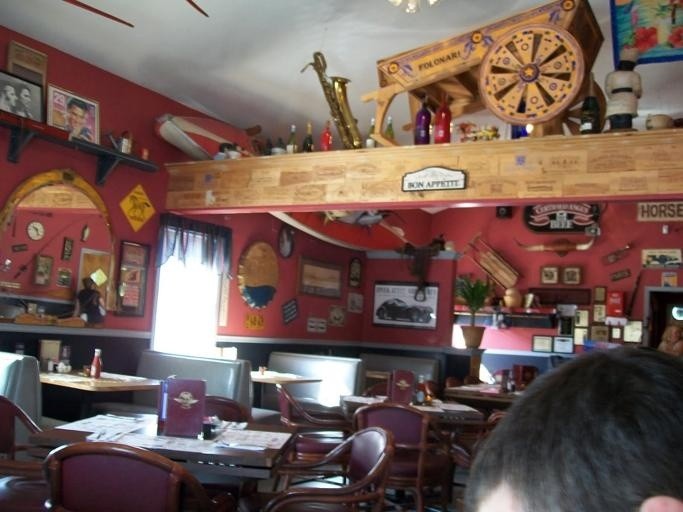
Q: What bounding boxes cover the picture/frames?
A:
[47,82,100,146]
[0,68,45,126]
[371,281,440,331]
[607,0,682,70]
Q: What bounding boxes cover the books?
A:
[386,369,414,405]
[157,378,207,438]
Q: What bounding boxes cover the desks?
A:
[446,380,530,403]
[38,367,162,392]
[367,370,392,381]
[252,369,323,386]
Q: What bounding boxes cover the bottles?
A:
[284,125,298,154]
[579,71,600,134]
[506,369,514,391]
[433,92,451,143]
[384,117,394,141]
[413,93,431,144]
[364,118,377,148]
[302,121,314,152]
[319,120,332,151]
[413,374,426,405]
[90,349,102,379]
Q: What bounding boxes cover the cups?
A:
[258,366,265,375]
[82,365,91,377]
[137,148,148,160]
[270,147,285,155]
[59,345,71,366]
[644,113,673,129]
[202,421,217,439]
[14,343,25,354]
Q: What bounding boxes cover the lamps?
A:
[386,0,439,16]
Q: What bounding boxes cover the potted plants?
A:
[449,272,495,350]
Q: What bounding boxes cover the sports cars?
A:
[375,297,434,324]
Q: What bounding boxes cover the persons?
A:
[64,97,93,142]
[463,346,683,512]
[13,83,34,120]
[0,80,18,113]
[603,47,641,133]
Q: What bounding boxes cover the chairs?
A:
[1,351,70,463]
[257,350,364,431]
[92,350,282,431]
[2,395,48,511]
[237,426,396,512]
[351,403,433,511]
[42,441,210,512]
[339,393,484,511]
[361,353,439,393]
[275,384,352,490]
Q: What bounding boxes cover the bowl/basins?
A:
[502,295,522,308]
